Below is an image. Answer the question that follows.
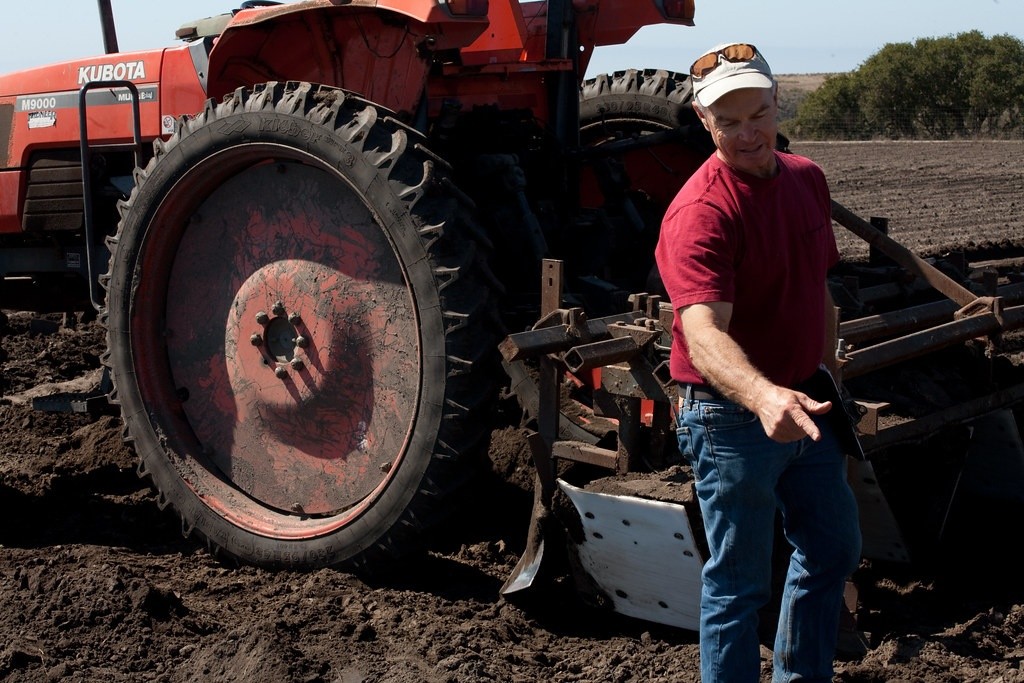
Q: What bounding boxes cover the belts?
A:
[677,380,729,401]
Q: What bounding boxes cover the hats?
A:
[689,42,772,107]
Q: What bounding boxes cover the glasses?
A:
[690,43,771,88]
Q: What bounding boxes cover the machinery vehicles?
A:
[0,0,1024,635]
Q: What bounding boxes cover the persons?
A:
[654,42,862,683]
